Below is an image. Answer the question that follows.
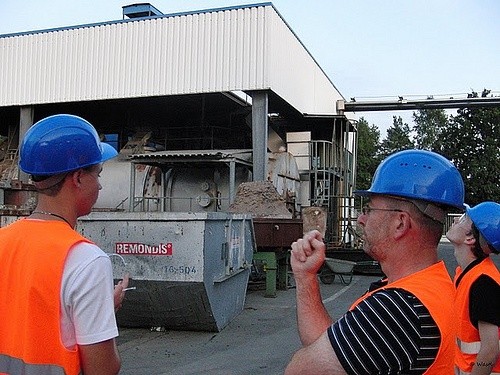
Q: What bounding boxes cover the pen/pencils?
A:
[122,287,137,291]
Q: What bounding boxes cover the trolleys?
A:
[320,257,380,287]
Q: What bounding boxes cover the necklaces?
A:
[31,210,73,229]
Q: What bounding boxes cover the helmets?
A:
[462,202,500,253]
[352,151,466,209]
[18,114,119,174]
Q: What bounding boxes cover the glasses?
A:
[458,213,478,238]
[361,206,402,215]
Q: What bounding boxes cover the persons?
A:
[0,114,129,375]
[281,150,465,375]
[445,202,500,375]
